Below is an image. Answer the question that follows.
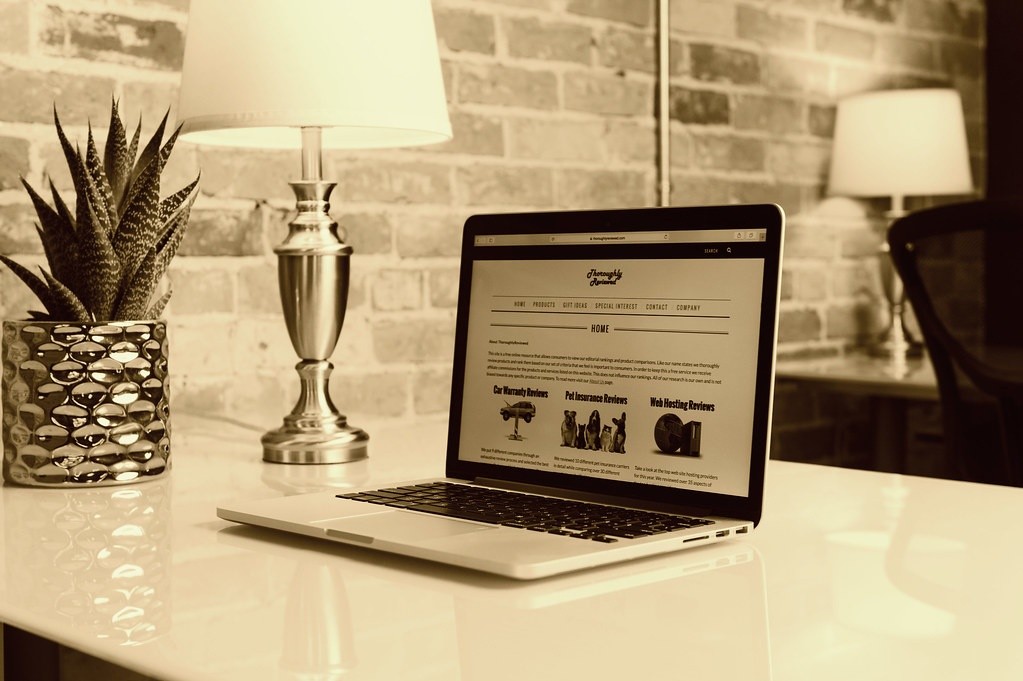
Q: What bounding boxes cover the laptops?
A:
[216,202,787,581]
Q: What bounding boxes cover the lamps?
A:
[821,80,976,361]
[173,0,453,467]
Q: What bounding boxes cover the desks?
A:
[0,410,1022,681]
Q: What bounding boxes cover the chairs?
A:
[884,199,1022,487]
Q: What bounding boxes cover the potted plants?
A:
[1,90,205,488]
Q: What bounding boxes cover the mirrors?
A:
[652,1,986,397]
